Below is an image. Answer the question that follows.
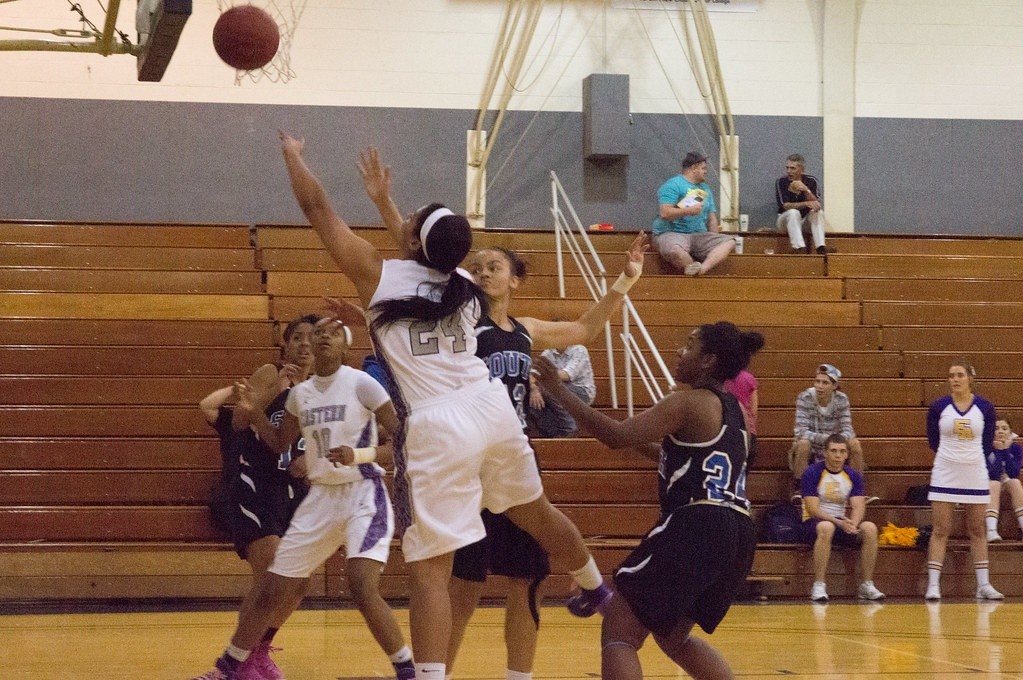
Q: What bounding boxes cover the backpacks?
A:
[762,502,802,543]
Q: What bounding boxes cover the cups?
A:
[735,237,744,254]
[740,214,748,231]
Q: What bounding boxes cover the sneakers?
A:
[858,579,885,600]
[257,642,285,680]
[214,648,265,680]
[568,584,614,618]
[811,582,829,601]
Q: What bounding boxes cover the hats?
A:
[817,364,841,381]
[682,151,712,167]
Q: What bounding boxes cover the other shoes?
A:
[816,246,827,255]
[976,586,1005,599]
[797,247,809,254]
[924,589,941,599]
[791,495,802,504]
[864,496,879,506]
[393,662,416,680]
[987,533,1003,543]
[685,262,701,276]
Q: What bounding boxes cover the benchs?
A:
[1,219,1023,598]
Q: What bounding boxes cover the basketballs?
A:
[213,6,280,69]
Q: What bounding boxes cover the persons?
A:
[278,127,613,680]
[530,321,765,680]
[190,317,415,680]
[788,364,880,505]
[925,359,1006,599]
[652,152,737,275]
[523,344,595,439]
[363,352,407,445]
[776,154,827,254]
[801,434,886,601]
[354,145,650,680]
[718,370,759,477]
[984,418,1023,543]
